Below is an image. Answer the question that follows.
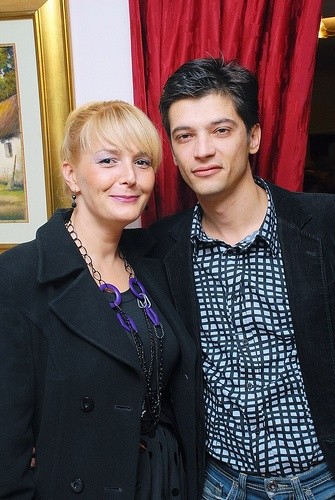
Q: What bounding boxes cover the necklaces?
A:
[64,220,170,439]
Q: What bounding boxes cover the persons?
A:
[1,100,241,500]
[136,50,335,499]
